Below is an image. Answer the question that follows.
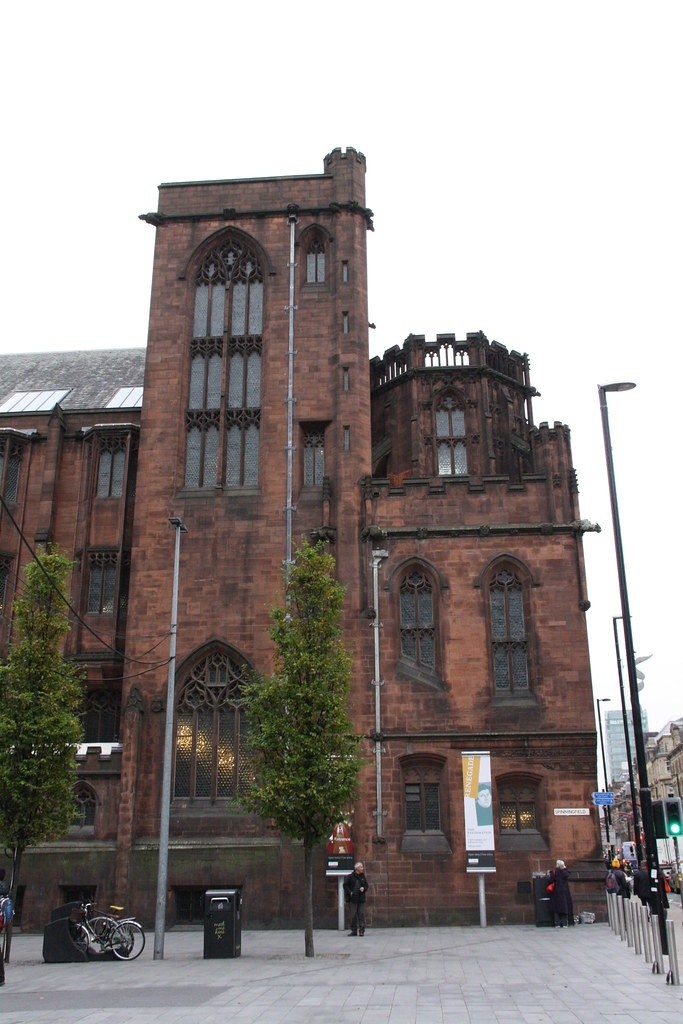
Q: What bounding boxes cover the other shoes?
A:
[348,932,357,936]
[563,926,567,929]
[359,930,364,937]
[556,925,560,929]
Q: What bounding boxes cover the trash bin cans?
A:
[203,888,243,959]
[532,878,554,927]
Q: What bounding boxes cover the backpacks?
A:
[606,870,621,894]
[0,897,13,925]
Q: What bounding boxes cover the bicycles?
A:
[73,901,146,961]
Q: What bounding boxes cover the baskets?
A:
[71,908,86,922]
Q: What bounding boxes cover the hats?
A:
[612,857,620,868]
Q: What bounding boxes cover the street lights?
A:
[599,382,670,955]
[612,615,643,868]
[596,698,618,860]
[152,516,191,961]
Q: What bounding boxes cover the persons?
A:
[0,878,12,986]
[546,859,575,927]
[605,848,683,922]
[343,862,368,936]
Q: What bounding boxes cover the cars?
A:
[624,856,683,894]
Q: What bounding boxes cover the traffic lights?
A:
[662,796,683,838]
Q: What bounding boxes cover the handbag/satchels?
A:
[547,882,554,893]
[664,876,671,893]
